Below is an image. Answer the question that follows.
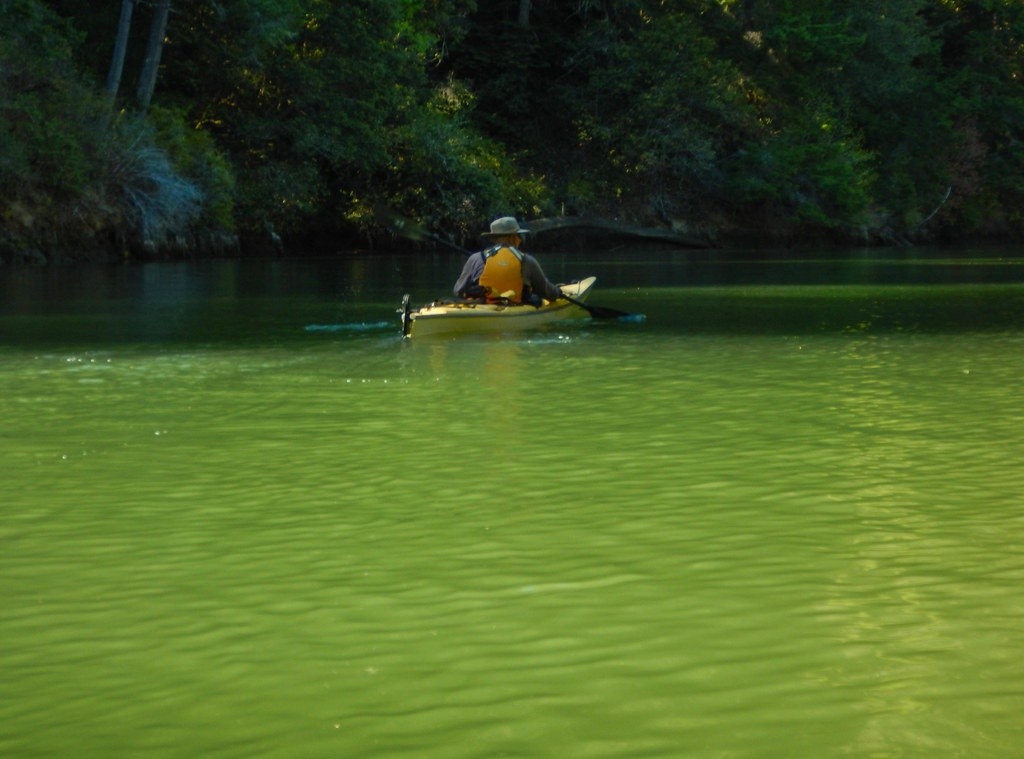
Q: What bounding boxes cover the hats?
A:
[480,217,530,236]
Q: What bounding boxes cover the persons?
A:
[454,217,562,309]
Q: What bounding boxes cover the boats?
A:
[396,274,597,342]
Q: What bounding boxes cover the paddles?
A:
[561,292,630,320]
